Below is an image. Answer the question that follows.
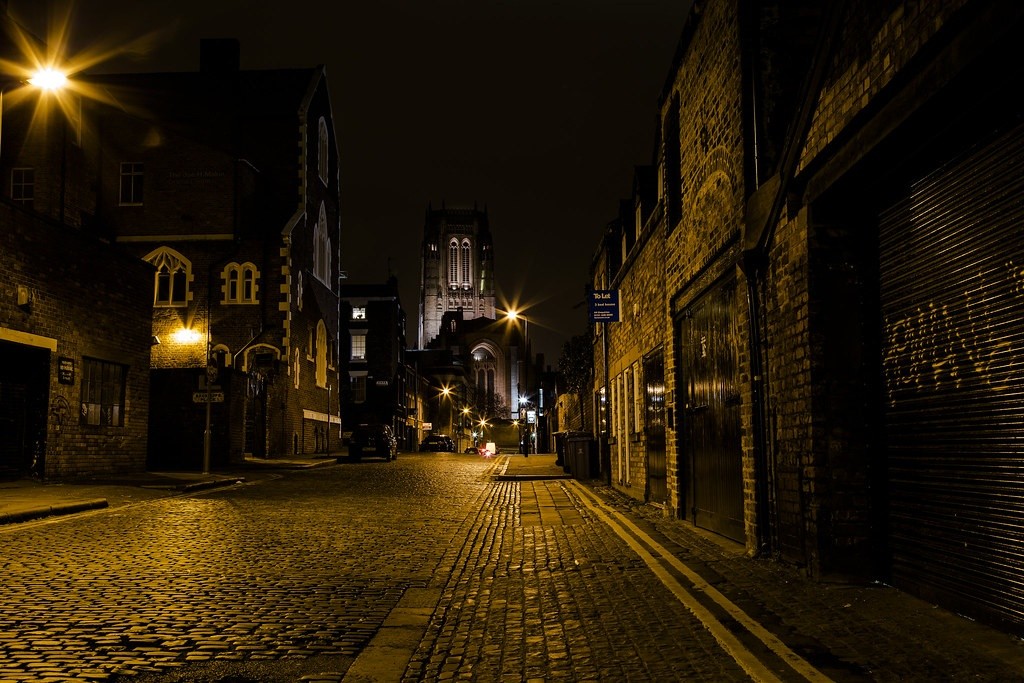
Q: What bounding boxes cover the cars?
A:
[348,424,399,463]
[422,434,456,453]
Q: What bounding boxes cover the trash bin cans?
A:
[551,429,594,479]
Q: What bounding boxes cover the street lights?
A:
[508,311,530,457]
